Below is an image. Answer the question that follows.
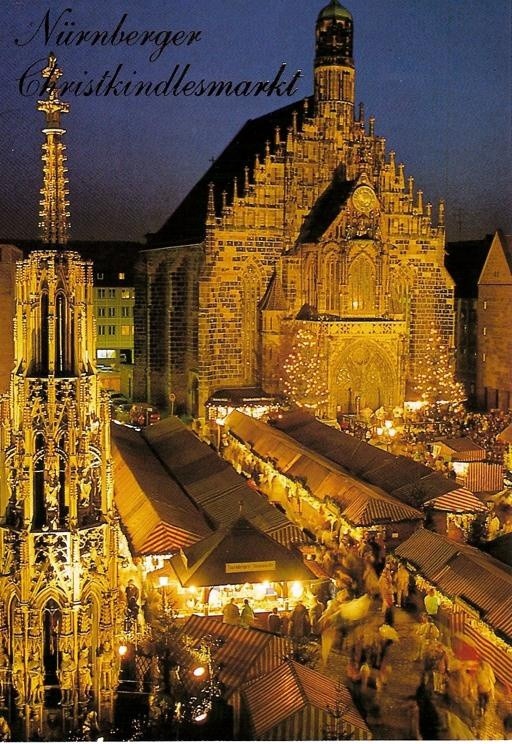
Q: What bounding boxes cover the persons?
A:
[222,543,512,739]
[123,579,140,630]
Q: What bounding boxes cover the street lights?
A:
[216,419,224,451]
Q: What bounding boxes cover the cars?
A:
[107,391,161,426]
